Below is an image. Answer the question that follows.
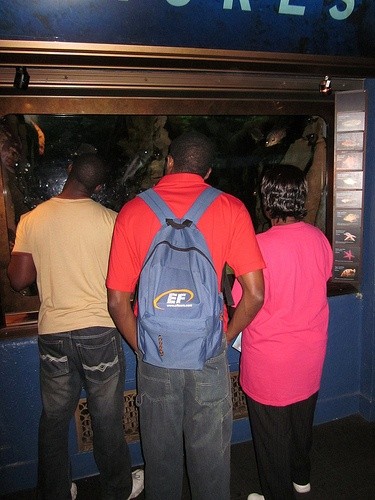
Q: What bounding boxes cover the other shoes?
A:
[292,482,311,493]
[247,493,265,500]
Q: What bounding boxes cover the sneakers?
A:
[126,469,144,500]
[70,482,77,500]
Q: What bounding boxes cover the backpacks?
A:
[136,187,224,371]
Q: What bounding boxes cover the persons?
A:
[7,155,135,500]
[106,130,267,500]
[228,164,334,500]
